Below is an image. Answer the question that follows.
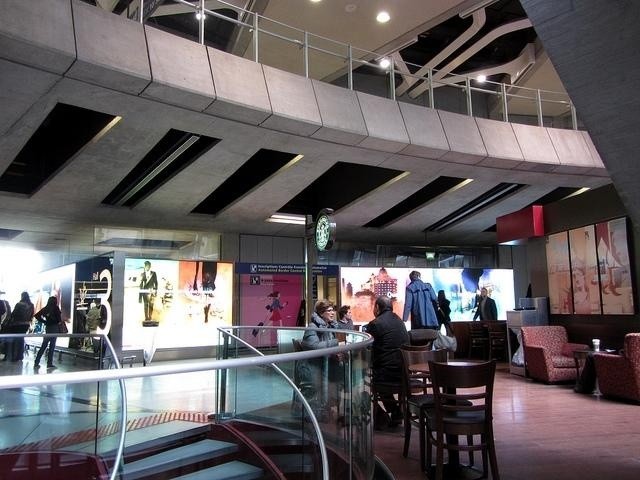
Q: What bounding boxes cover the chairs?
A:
[467,320,515,366]
[519,324,589,383]
[292,339,432,424]
[400,346,448,459]
[421,361,499,478]
[593,332,640,401]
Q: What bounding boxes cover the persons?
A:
[364,296,410,431]
[473,287,498,320]
[295,300,351,420]
[434,290,450,323]
[338,305,353,331]
[261,291,287,335]
[139,261,157,320]
[34,296,62,368]
[6,292,34,361]
[567,258,591,315]
[403,271,440,329]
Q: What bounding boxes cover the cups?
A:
[592,339,600,352]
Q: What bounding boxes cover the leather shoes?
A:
[374,414,392,431]
[391,412,403,425]
[47,364,55,367]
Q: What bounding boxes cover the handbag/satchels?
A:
[58,322,68,333]
[434,330,457,353]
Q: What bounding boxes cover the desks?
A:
[573,348,614,386]
[408,359,487,479]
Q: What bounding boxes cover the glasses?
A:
[325,310,334,312]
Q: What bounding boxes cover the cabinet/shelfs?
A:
[506,297,548,377]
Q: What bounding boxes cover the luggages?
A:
[252,311,272,337]
